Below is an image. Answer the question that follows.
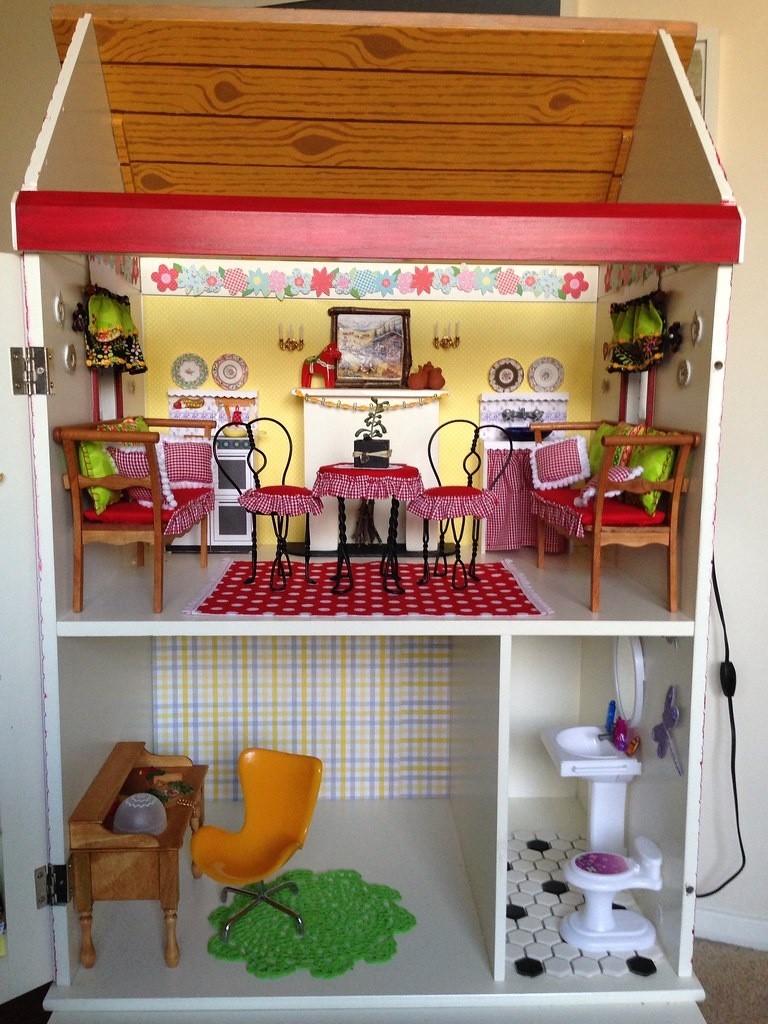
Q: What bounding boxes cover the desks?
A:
[311,461,423,594]
[69,741,208,969]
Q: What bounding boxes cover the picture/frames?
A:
[328,307,412,390]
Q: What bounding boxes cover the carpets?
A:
[207,869,415,981]
[182,557,555,620]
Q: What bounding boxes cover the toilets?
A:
[558,834,663,952]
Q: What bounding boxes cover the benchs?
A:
[531,419,701,612]
[54,415,216,613]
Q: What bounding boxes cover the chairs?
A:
[191,748,322,942]
[212,418,323,590]
[405,421,513,591]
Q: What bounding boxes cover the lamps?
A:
[279,321,304,350]
[434,318,460,348]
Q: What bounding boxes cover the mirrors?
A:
[612,635,644,729]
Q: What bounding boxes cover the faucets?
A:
[520,407,528,420]
[598,723,614,741]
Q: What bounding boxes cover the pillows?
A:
[159,432,214,489]
[78,416,151,515]
[586,421,679,518]
[105,443,178,510]
[530,435,590,492]
[575,466,642,508]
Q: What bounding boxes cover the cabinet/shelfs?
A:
[167,450,253,552]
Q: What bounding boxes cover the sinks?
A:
[556,725,619,759]
[507,427,553,441]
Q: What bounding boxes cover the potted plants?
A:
[352,397,392,468]
